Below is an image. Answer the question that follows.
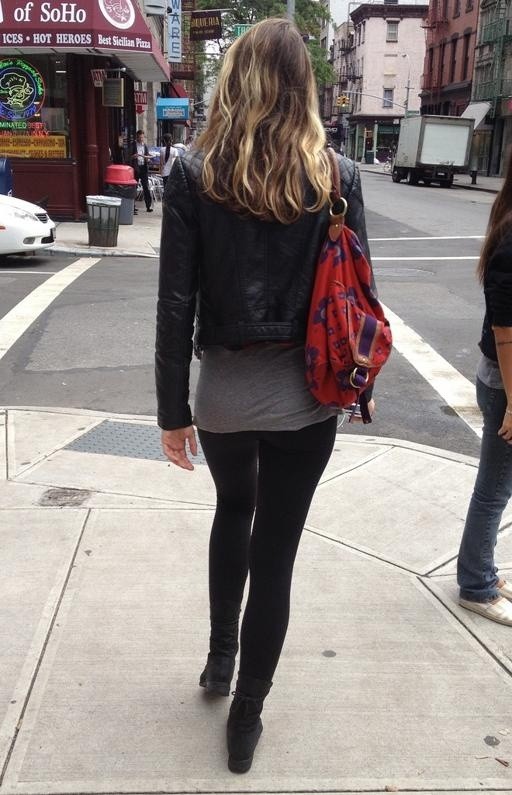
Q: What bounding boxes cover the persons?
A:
[160,132,179,187]
[152,16,379,775]
[124,130,153,213]
[456,150,512,627]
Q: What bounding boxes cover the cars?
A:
[0,194,56,255]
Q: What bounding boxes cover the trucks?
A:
[391,114,475,185]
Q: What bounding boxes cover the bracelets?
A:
[504,410,512,414]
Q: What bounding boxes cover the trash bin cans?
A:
[85,195,121,246]
[103,164,138,225]
[366,150,374,164]
[0,157,13,196]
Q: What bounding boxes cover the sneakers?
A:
[496,576,512,598]
[459,593,512,626]
[147,207,153,212]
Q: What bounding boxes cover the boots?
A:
[227,671,274,774]
[200,605,241,696]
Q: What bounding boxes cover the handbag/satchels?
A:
[305,194,392,410]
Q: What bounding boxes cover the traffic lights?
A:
[336,96,350,107]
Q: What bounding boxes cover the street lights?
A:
[401,53,415,118]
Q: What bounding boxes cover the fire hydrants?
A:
[469,167,478,184]
